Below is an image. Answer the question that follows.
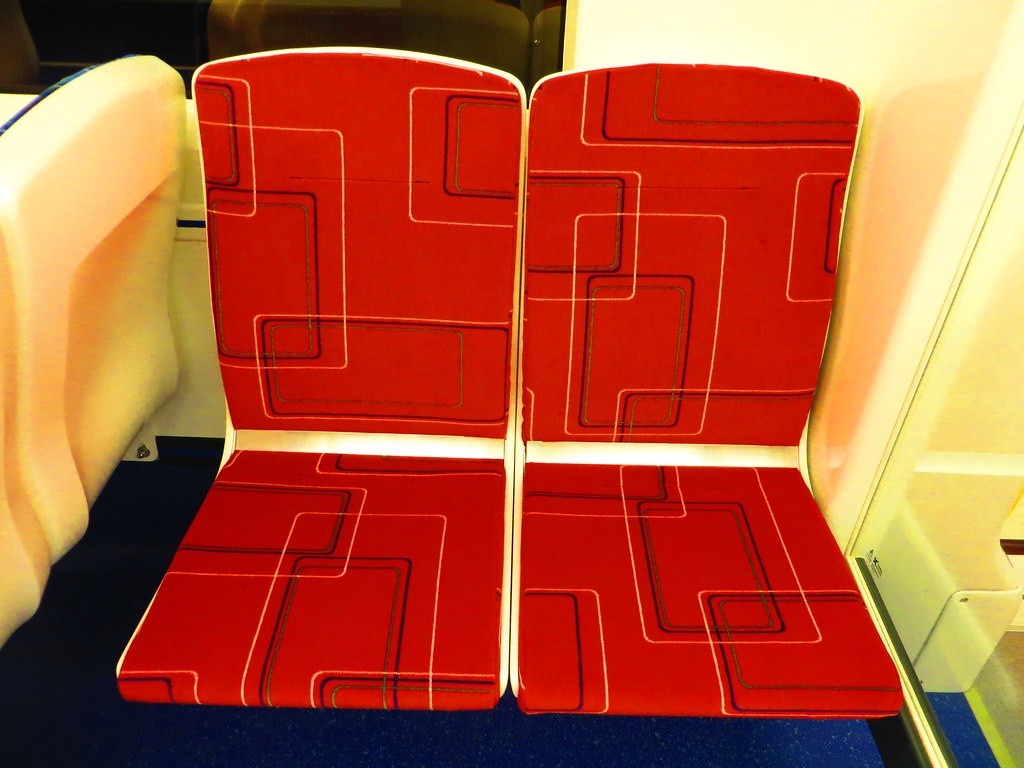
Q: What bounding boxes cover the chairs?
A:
[115,50,523,712]
[0,54,189,653]
[511,61,905,723]
